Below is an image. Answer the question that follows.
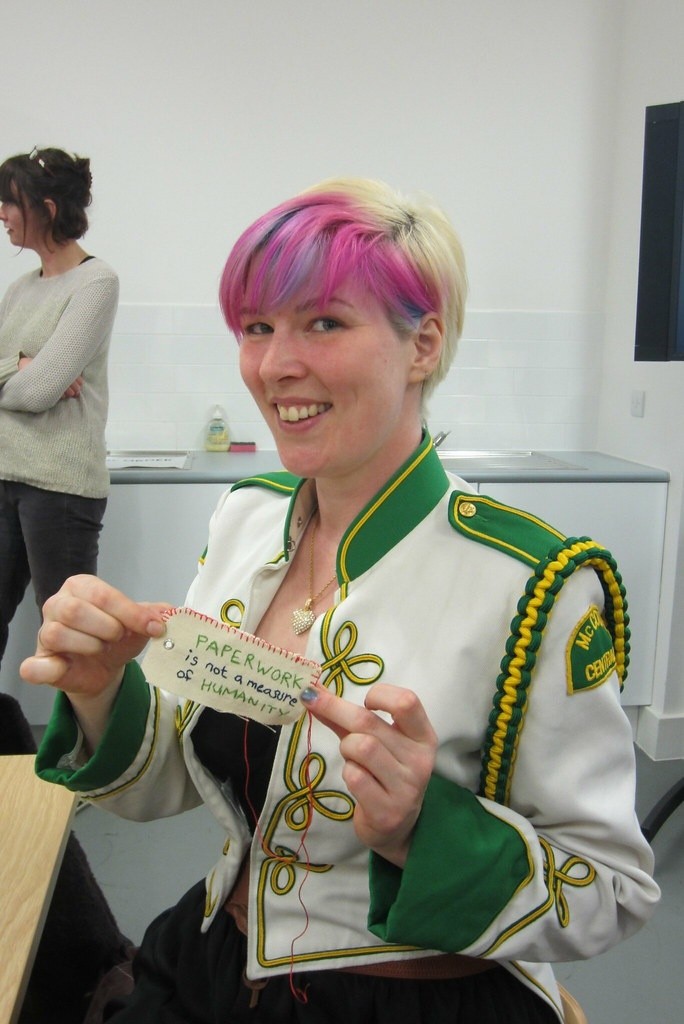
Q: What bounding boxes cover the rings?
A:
[37,624,45,650]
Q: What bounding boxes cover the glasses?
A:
[29,145,53,174]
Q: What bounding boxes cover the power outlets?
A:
[629,388,646,418]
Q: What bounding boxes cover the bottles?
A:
[204,404,231,452]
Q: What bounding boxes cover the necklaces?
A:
[292,517,338,635]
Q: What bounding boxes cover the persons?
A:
[0,147,119,754]
[17,172,663,1024]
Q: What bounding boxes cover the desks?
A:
[0,755,80,1024]
[0,448,671,741]
